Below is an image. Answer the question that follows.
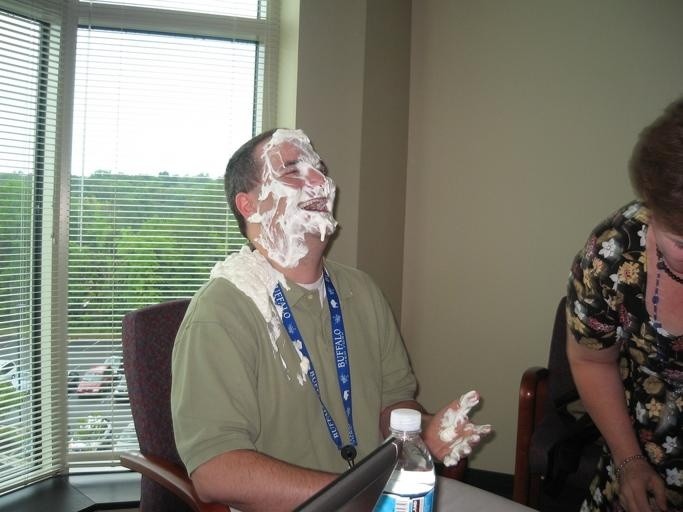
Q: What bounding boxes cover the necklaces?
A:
[645,238,682,288]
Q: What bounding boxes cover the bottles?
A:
[368,409,437,511]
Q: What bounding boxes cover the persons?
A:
[545,99,680,512]
[170,131,492,510]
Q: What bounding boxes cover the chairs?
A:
[120,297,468,512]
[513,296,601,510]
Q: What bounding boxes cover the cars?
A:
[0,352,142,454]
